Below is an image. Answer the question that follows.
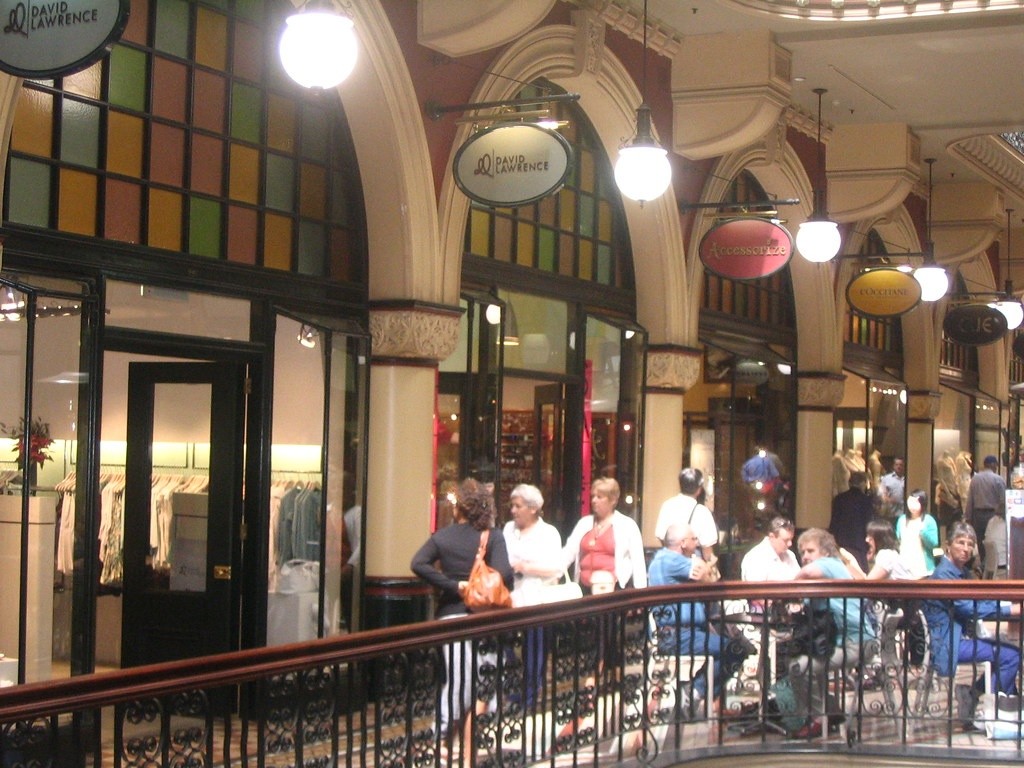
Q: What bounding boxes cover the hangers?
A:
[54,469,322,499]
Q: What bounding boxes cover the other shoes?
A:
[794,707,847,739]
[680,685,700,721]
[862,674,881,691]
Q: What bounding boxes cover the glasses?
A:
[775,520,793,529]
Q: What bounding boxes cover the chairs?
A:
[840,609,993,742]
[642,607,717,730]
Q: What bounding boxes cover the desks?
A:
[983,610,1024,738]
[707,610,805,734]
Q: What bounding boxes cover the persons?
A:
[908,521,1024,730]
[740,515,804,615]
[549,476,647,699]
[839,520,918,689]
[502,484,562,718]
[784,527,877,738]
[965,455,1007,580]
[828,472,875,576]
[648,523,748,721]
[654,468,718,563]
[833,449,882,494]
[935,450,973,554]
[410,477,514,768]
[877,456,905,529]
[895,489,939,581]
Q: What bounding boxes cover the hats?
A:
[984,455,998,464]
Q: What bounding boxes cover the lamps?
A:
[612,0,673,204]
[908,159,949,302]
[985,210,1024,333]
[796,87,842,264]
[279,1,356,89]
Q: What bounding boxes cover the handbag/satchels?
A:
[772,677,807,730]
[464,527,513,613]
[981,692,1024,741]
[791,610,837,655]
[529,561,583,606]
